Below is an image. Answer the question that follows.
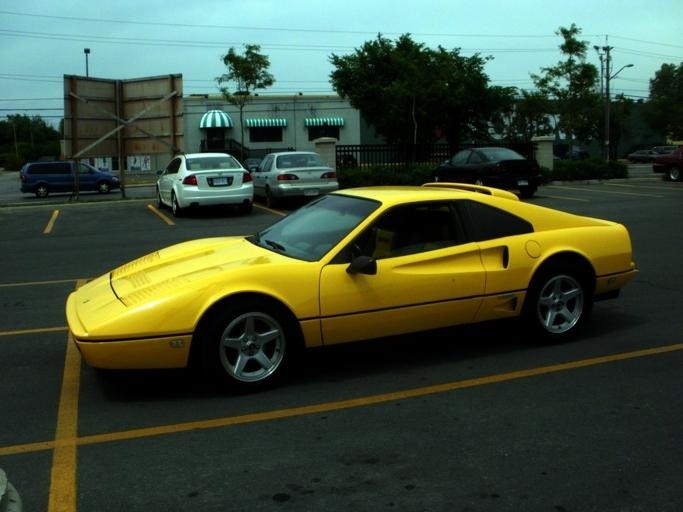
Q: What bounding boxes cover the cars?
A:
[155,153,254,217]
[243,150,360,209]
[562,150,591,161]
[432,147,541,198]
[628,145,683,182]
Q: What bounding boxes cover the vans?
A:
[20,161,121,198]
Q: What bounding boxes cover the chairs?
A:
[351,214,426,256]
[187,161,232,172]
[283,160,318,168]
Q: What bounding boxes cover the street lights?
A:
[603,45,633,163]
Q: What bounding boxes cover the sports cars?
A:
[65,181,641,397]
